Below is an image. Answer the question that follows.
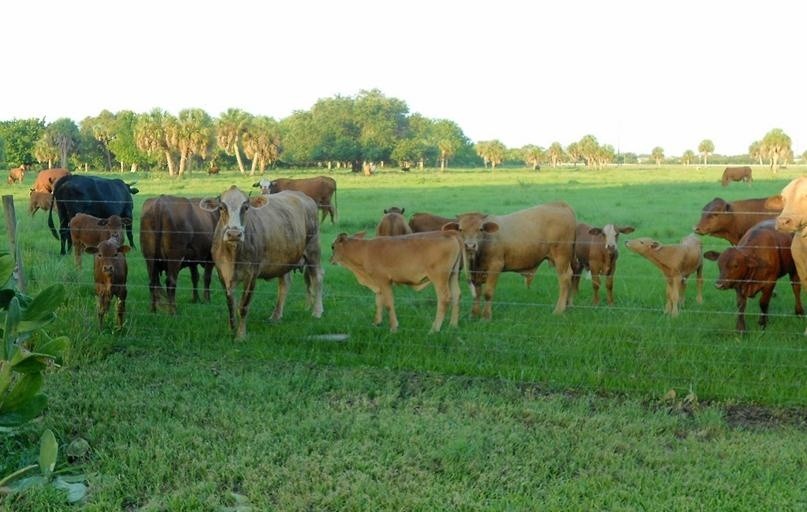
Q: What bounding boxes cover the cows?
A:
[83,239,132,331]
[441,200,576,321]
[721,166,752,186]
[7,163,70,218]
[140,194,220,316]
[253,175,336,223]
[408,212,487,285]
[625,234,703,318]
[376,207,413,236]
[47,173,140,255]
[571,223,635,306]
[197,184,325,342]
[329,230,477,335]
[693,174,807,335]
[67,212,132,270]
